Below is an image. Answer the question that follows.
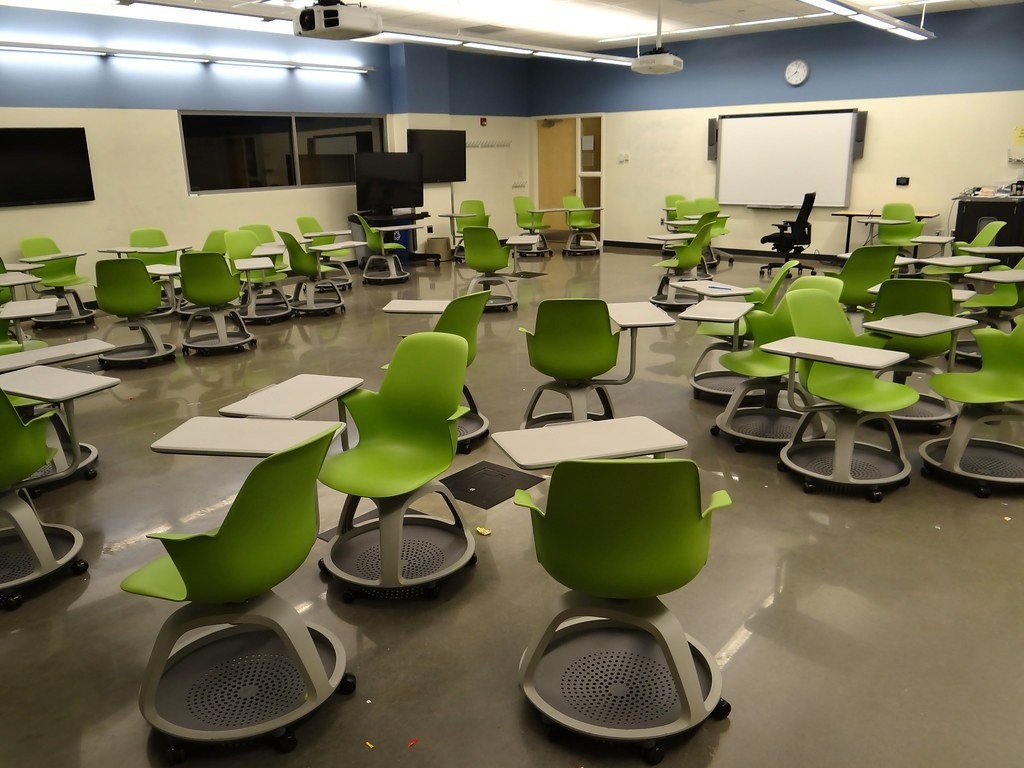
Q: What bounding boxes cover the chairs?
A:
[0,192,1024,768]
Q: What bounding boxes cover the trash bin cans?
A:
[393,221,414,261]
[426,237,454,261]
[347,212,388,270]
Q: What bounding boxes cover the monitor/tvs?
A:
[0,127,95,208]
[354,151,423,215]
[407,129,466,183]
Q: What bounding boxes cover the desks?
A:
[832,210,940,267]
[949,189,1024,285]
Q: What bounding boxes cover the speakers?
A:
[708,118,717,160]
[853,111,868,158]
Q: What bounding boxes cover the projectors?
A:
[293,5,382,41]
[631,53,683,74]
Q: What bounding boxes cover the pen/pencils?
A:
[708,286,731,290]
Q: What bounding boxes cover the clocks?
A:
[785,58,810,86]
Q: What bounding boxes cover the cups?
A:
[1016,181,1024,196]
[1011,183,1017,196]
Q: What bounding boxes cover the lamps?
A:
[380,26,633,67]
[800,1,937,44]
